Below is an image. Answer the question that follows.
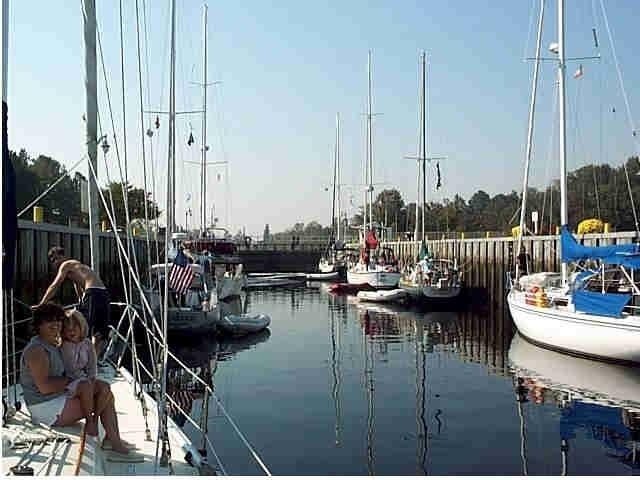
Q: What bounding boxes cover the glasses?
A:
[41,314,65,321]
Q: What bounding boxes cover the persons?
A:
[20,246,134,454]
[195,249,213,301]
[358,248,368,272]
[367,228,379,248]
[517,246,531,275]
[422,256,434,286]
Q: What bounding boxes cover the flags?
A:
[168,248,196,296]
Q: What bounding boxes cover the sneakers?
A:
[101,439,144,462]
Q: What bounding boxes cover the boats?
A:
[398,47,464,299]
[133,0,246,332]
[318,110,355,279]
[2,0,274,476]
[505,0,640,362]
[507,331,640,476]
[347,49,401,288]
[216,312,271,337]
[357,288,406,303]
[247,271,341,289]
